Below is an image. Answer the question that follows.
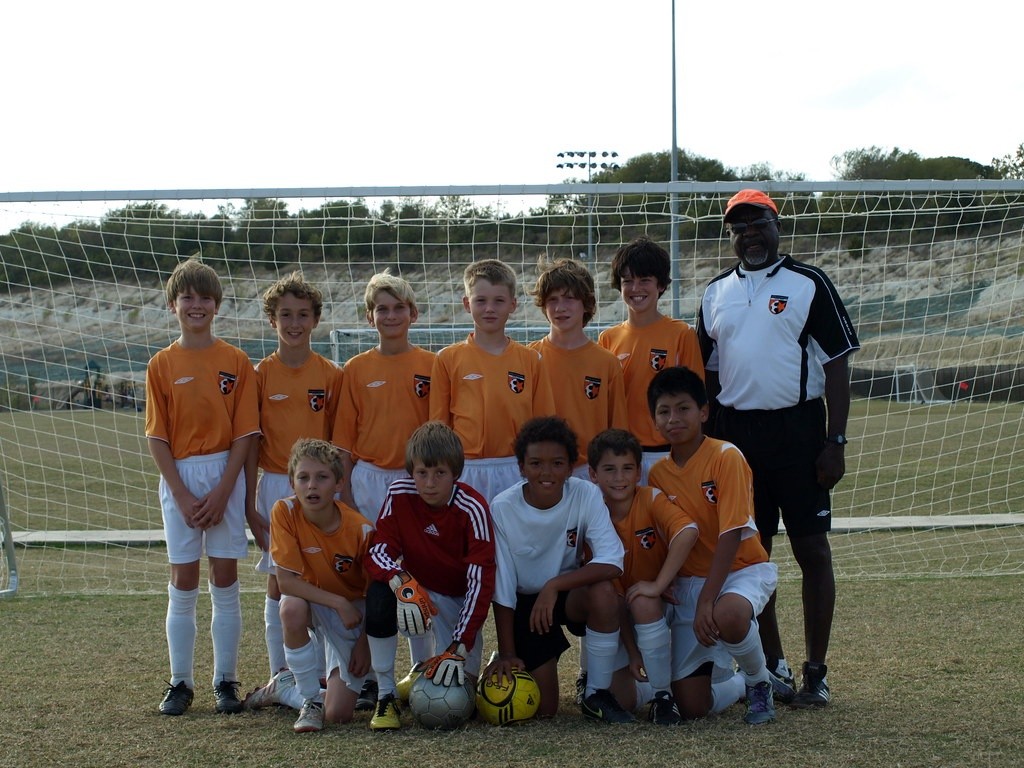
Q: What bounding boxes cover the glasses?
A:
[728,219,777,234]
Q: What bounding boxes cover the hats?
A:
[726,189,777,218]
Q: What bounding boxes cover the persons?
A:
[481,414,626,724]
[693,188,861,710]
[332,272,436,707]
[598,239,708,490]
[358,421,496,731]
[429,259,555,699]
[583,427,699,728]
[143,261,261,718]
[526,261,630,707]
[269,439,376,732]
[252,276,352,689]
[645,365,782,723]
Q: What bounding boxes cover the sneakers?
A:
[745,680,775,723]
[294,698,324,731]
[575,673,588,707]
[355,679,378,708]
[582,688,631,723]
[159,679,195,714]
[396,663,423,701]
[735,665,795,702]
[370,694,401,729]
[242,667,297,710]
[768,667,796,693]
[214,673,242,713]
[791,661,830,707]
[649,690,680,725]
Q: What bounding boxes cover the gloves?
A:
[417,641,466,687]
[389,570,438,636]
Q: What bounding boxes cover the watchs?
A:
[827,433,849,444]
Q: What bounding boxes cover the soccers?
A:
[475,665,540,725]
[409,670,476,729]
